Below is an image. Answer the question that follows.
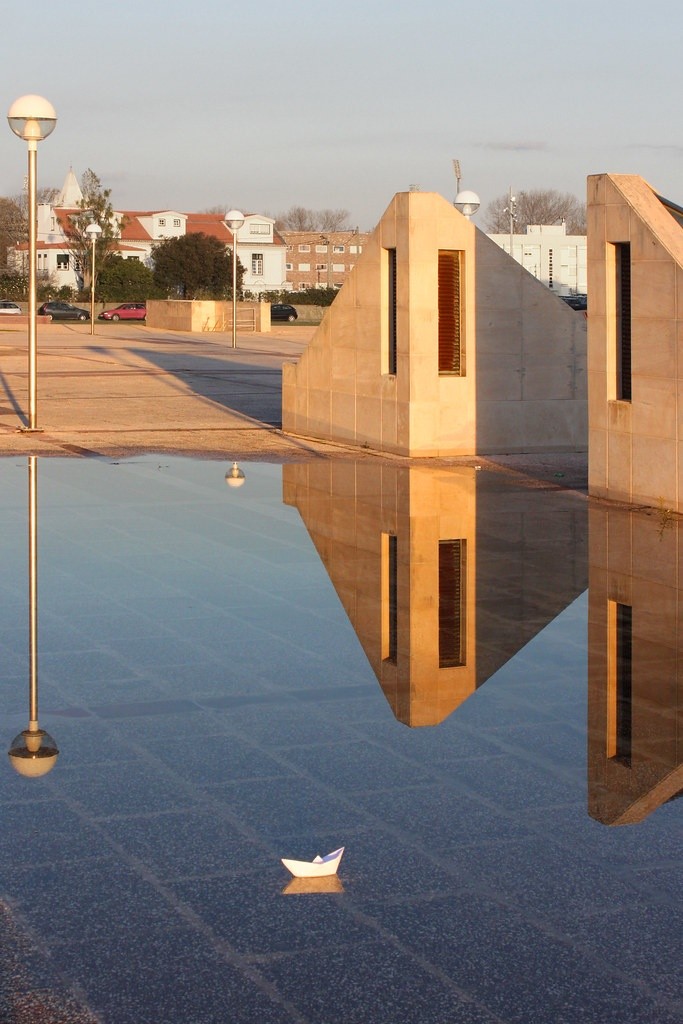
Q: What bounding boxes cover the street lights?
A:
[225,460,246,488]
[85,224,103,336]
[6,93,59,436]
[452,158,463,195]
[224,209,246,347]
[6,452,62,778]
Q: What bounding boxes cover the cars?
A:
[0,302,21,316]
[558,292,587,311]
[270,303,298,322]
[37,301,91,321]
[98,304,147,321]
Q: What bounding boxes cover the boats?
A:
[280,845,347,877]
[279,874,346,894]
